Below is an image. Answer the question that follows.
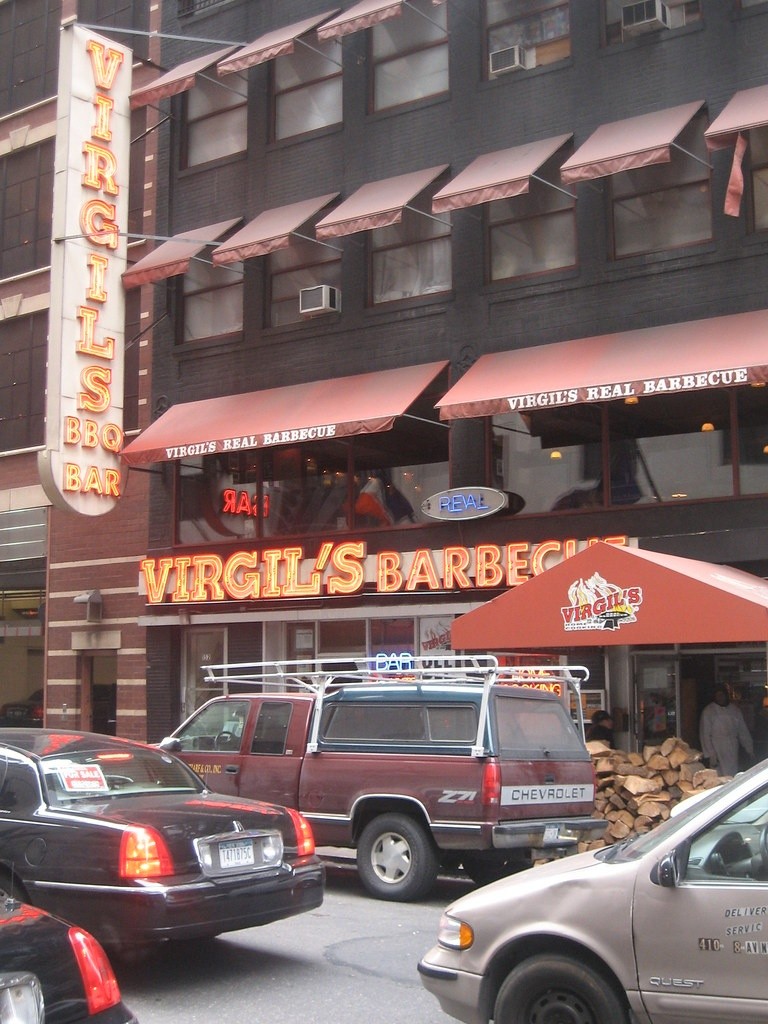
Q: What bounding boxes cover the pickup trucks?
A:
[160,654,608,903]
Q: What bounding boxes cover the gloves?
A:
[709,756,720,767]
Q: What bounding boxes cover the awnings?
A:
[433,310,767,421]
[316,0,479,46]
[210,190,344,269]
[430,132,580,217]
[121,215,245,290]
[702,84,767,140]
[314,161,453,242]
[216,8,342,79]
[118,359,455,474]
[129,40,248,121]
[450,541,768,650]
[559,100,715,186]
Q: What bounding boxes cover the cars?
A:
[416,761,767,1023]
[1,683,118,733]
[0,728,324,1024]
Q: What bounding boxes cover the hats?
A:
[592,710,610,723]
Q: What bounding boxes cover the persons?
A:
[698,683,759,777]
[586,710,617,749]
